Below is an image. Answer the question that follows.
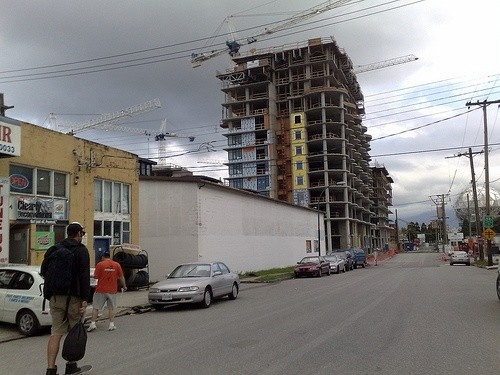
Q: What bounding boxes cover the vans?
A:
[336,248,366,269]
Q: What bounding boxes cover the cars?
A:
[0,266,103,337]
[294,255,331,278]
[147,262,240,311]
[447,250,473,266]
[322,255,347,274]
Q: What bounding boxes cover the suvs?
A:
[329,251,353,271]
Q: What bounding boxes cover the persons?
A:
[87,250,126,332]
[40,222,93,375]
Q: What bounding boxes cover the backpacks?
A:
[45,245,81,292]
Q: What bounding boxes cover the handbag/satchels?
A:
[62,318,87,361]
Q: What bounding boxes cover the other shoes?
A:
[66,365,93,375]
[87,326,96,332]
[108,326,116,330]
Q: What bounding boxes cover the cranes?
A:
[352,55,418,77]
[41,98,160,136]
[59,118,195,166]
[191,0,354,69]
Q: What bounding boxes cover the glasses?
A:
[80,231,85,235]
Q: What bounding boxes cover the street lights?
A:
[318,182,345,256]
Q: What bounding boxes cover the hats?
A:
[100,251,110,258]
[67,224,83,235]
